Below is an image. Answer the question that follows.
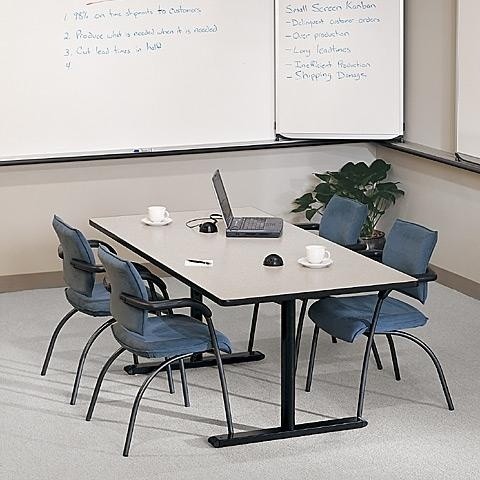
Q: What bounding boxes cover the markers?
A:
[134,148,152,152]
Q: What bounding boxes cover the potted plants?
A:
[290,159,404,262]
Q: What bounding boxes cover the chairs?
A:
[305,219,454,419]
[40,214,175,405]
[86,243,234,456]
[247,193,383,377]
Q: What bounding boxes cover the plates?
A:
[297,256,334,269]
[141,216,173,226]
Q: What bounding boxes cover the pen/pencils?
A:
[187,259,211,264]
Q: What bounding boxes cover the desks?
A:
[89,207,419,447]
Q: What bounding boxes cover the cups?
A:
[305,245,330,264]
[148,206,168,222]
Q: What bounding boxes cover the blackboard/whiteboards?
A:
[0,1,313,166]
[274,0,405,142]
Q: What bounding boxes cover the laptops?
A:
[211,168,284,238]
